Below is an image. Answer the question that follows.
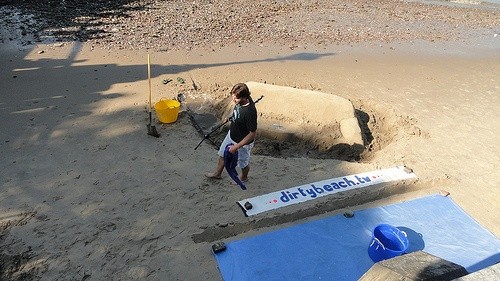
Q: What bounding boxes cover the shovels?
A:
[146,53,160,134]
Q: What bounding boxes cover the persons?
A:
[203,83,257,184]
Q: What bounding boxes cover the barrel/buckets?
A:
[155,97,180,124]
[369,224,409,263]
